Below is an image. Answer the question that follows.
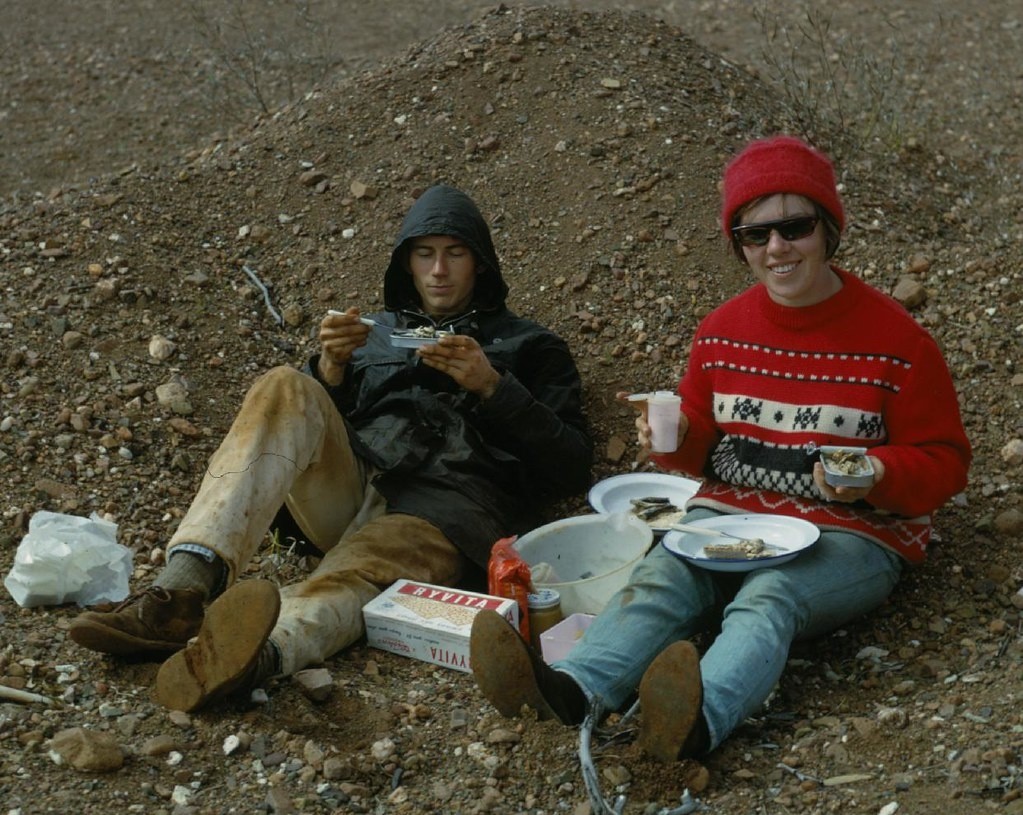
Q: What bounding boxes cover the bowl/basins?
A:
[539,614,596,665]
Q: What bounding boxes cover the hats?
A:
[722,136,843,239]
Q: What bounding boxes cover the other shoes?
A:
[470,610,585,725]
[637,640,703,763]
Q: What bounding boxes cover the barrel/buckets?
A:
[511,514,655,619]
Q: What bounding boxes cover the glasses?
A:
[731,216,821,246]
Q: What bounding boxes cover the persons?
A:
[70,187,591,712]
[470,135,972,768]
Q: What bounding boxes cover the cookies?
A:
[390,594,480,626]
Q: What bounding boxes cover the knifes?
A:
[669,522,789,551]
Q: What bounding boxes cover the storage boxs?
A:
[539,612,598,665]
[362,578,520,675]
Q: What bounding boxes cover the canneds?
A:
[525,588,563,653]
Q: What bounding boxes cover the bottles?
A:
[526,589,562,652]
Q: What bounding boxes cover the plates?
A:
[662,513,822,573]
[820,451,875,488]
[588,472,701,535]
[389,329,451,348]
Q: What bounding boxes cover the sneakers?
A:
[70,586,206,661]
[156,580,280,713]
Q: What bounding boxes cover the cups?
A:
[647,395,680,453]
